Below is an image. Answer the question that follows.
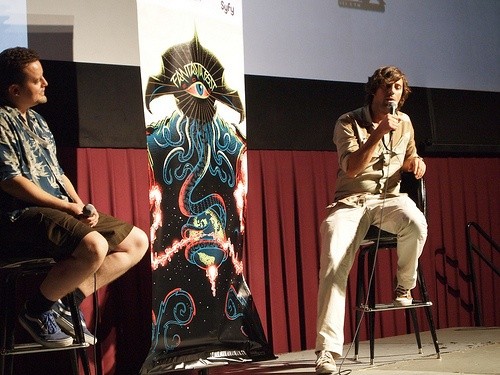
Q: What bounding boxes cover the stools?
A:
[354,171,441,365]
[0,245,102,375]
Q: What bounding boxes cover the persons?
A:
[314,66,428,373]
[0,46,149,349]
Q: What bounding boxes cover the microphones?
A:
[83,203,95,217]
[388,101,398,140]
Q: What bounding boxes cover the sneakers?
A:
[391,276,413,308]
[17,298,97,349]
[314,350,337,374]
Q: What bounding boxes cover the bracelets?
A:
[413,156,424,162]
